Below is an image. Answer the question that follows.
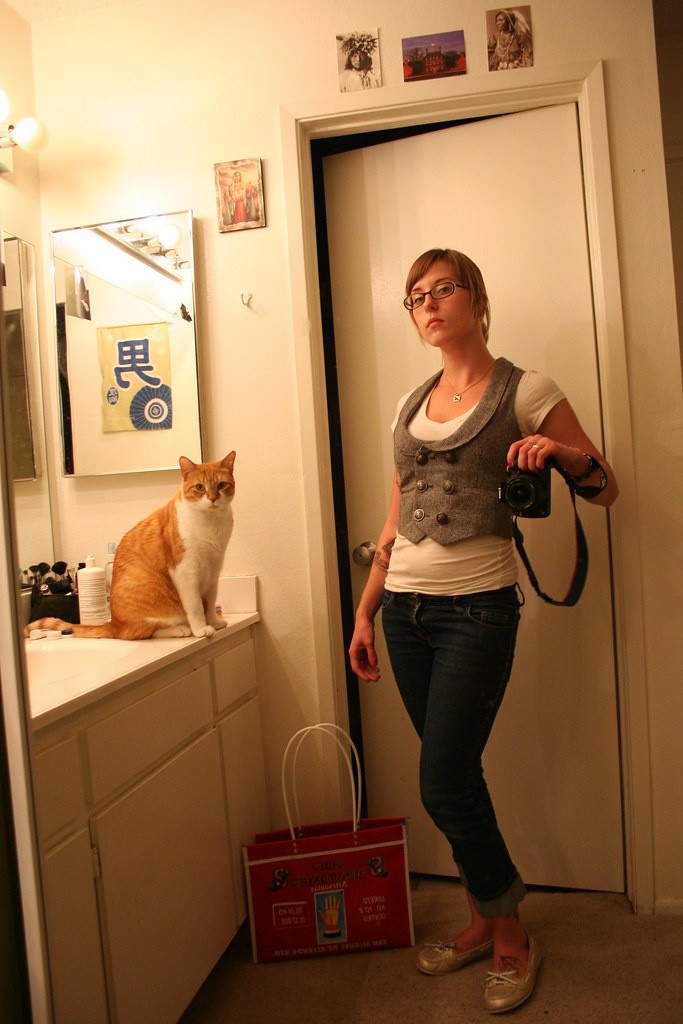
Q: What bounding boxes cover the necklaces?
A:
[498,33,514,57]
[443,360,494,402]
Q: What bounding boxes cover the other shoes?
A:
[483,930,541,1014]
[415,936,493,977]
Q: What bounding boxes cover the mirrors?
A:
[49,209,204,479]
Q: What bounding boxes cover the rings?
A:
[533,444,542,449]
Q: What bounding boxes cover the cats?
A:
[24,449,236,642]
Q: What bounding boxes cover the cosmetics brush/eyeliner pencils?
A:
[24,561,77,596]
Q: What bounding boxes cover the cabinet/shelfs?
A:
[33,637,271,1024]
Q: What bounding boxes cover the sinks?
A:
[21,638,141,687]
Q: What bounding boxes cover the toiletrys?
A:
[106,543,115,627]
[77,554,107,626]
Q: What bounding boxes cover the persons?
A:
[339,33,381,92]
[348,248,618,1013]
[487,9,533,70]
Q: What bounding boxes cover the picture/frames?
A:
[212,156,266,235]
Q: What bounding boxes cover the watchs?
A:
[567,454,601,481]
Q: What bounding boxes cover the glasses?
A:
[404,281,469,310]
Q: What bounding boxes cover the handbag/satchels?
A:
[241,723,415,963]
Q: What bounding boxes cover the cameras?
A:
[497,456,552,519]
